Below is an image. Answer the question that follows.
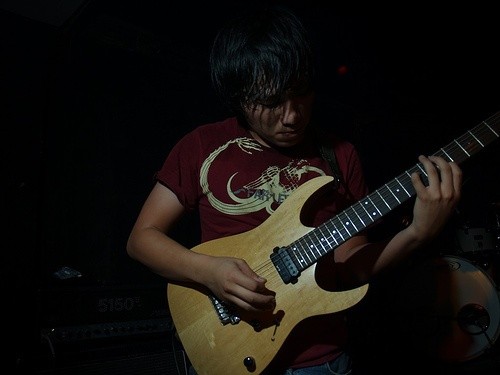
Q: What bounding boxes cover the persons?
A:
[126,15,463,375]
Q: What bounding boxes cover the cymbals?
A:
[477,203,500,209]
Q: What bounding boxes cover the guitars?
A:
[165,111,500,375]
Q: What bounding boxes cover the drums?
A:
[437,254,500,363]
[453,225,496,253]
